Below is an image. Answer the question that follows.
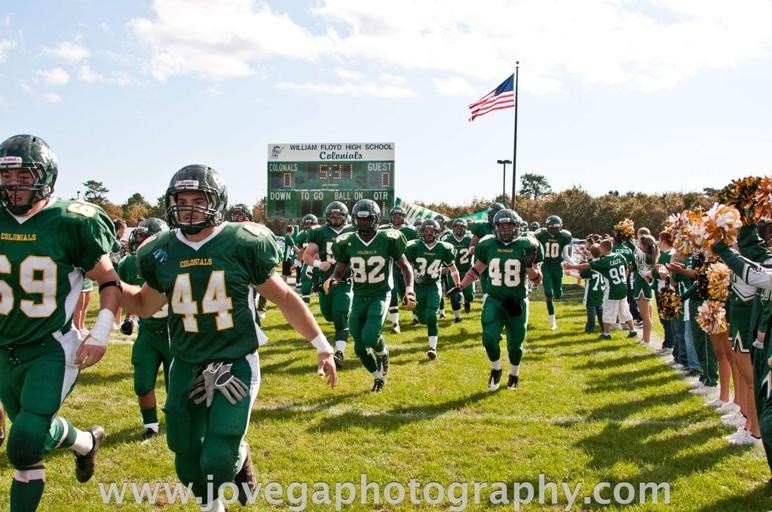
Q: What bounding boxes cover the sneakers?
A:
[655,342,764,447]
[371,376,386,394]
[121,318,133,335]
[377,345,390,375]
[595,319,650,346]
[487,369,503,391]
[390,300,472,334]
[73,425,107,482]
[138,428,158,445]
[428,347,437,360]
[236,442,256,506]
[333,351,344,368]
[550,324,558,330]
[507,374,520,390]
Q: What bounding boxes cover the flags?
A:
[469,71,517,121]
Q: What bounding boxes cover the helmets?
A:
[164,164,228,235]
[230,203,253,224]
[0,134,59,215]
[286,198,564,243]
[127,216,169,254]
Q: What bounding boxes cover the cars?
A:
[569,237,588,245]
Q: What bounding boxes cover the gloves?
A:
[322,273,341,296]
[521,245,537,268]
[446,285,461,298]
[214,362,250,406]
[188,362,215,408]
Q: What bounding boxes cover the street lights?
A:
[496,158,514,203]
